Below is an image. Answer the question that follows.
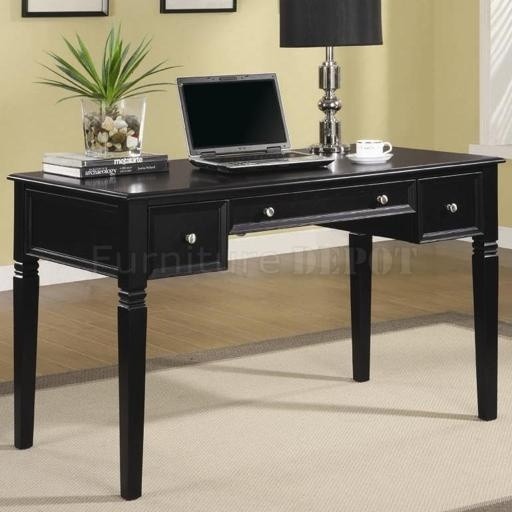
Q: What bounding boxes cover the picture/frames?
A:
[21,1,110,17]
[160,0,238,13]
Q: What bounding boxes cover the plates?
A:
[346,153,394,165]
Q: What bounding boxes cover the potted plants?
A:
[33,27,184,158]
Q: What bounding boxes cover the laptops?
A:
[177,72,335,177]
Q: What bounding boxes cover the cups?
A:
[356,140,393,157]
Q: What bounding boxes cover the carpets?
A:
[0,309,512,511]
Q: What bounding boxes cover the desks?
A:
[6,145,508,501]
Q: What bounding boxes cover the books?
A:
[42,162,169,178]
[41,153,168,168]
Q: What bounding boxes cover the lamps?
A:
[278,1,385,154]
